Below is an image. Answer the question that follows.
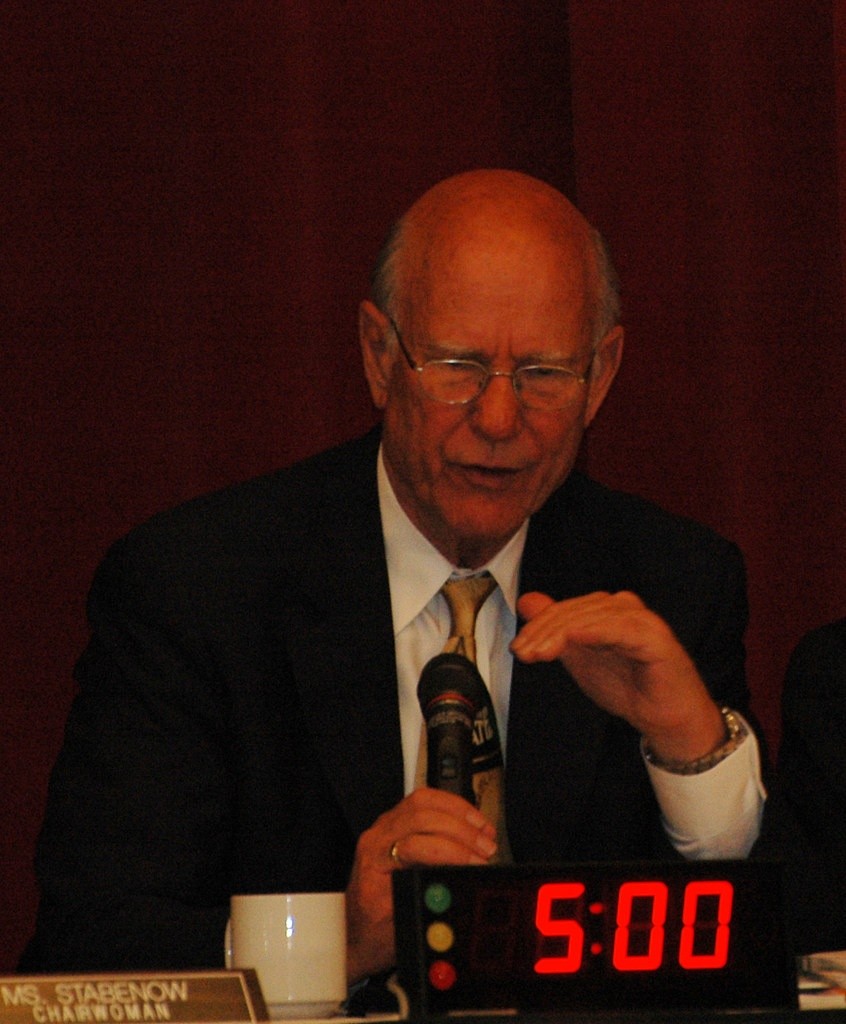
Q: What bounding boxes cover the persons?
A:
[13,168,766,1014]
[776,615,846,956]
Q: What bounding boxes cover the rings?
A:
[391,839,413,871]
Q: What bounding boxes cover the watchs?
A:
[641,706,748,775]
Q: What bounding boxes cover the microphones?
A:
[417,651,487,812]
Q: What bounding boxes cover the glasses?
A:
[389,316,598,410]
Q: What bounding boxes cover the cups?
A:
[224,892,349,1017]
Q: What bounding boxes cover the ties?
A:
[415,576,501,868]
